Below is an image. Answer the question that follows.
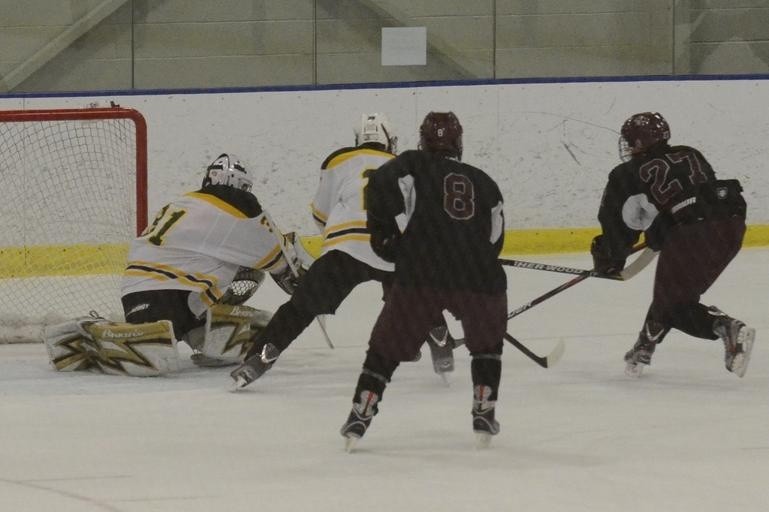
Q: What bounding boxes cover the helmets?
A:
[354,113,393,150]
[618,112,670,162]
[419,112,463,160]
[202,153,253,192]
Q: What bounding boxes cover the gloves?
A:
[370,232,402,261]
[590,236,625,273]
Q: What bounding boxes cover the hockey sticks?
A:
[505,331,568,368]
[497,245,659,281]
[263,206,335,350]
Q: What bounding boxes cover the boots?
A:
[426,325,455,373]
[471,385,500,434]
[706,305,745,371]
[625,321,665,367]
[230,341,280,386]
[341,389,378,438]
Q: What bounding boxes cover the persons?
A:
[118,153,324,382]
[230,113,455,387]
[590,110,748,371]
[341,110,508,435]
